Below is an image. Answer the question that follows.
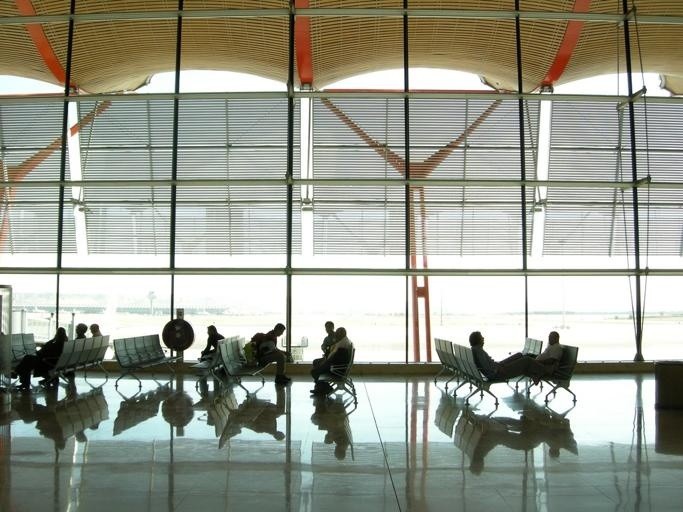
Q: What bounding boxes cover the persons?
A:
[198,324,225,362]
[88,323,102,337]
[468,330,558,382]
[3,326,68,390]
[74,431,87,442]
[73,322,87,338]
[310,320,337,366]
[254,323,293,386]
[468,424,553,474]
[532,331,563,366]
[238,381,288,442]
[544,436,563,459]
[312,395,349,461]
[8,400,66,450]
[308,326,353,394]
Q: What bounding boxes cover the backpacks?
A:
[244,333,265,366]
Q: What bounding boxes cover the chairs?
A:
[188,337,357,406]
[36,391,357,461]
[434,397,581,461]
[433,336,579,407]
[9,334,182,390]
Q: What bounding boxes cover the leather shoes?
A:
[275,374,291,384]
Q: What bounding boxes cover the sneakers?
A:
[38,376,59,385]
[16,383,29,389]
[5,372,17,379]
